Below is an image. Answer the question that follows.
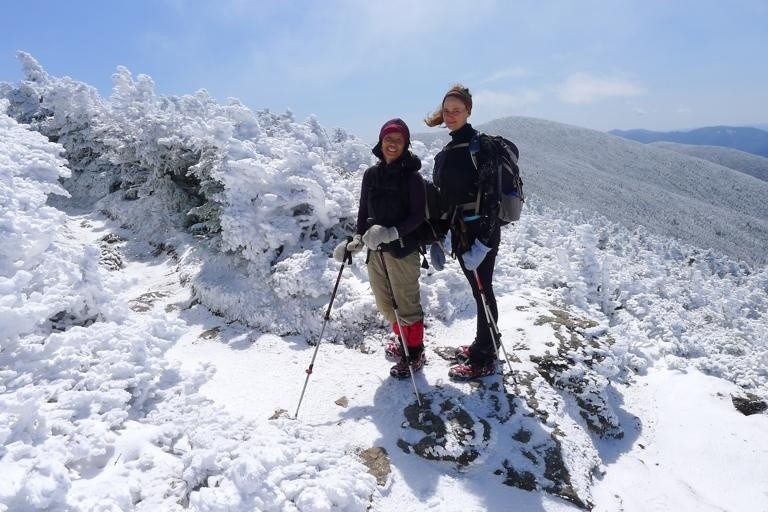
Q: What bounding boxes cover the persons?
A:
[332,117,427,378]
[431,86,501,381]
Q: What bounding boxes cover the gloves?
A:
[333,233,365,262]
[361,224,399,251]
[430,243,445,271]
[461,238,493,271]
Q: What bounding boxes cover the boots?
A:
[385,334,427,378]
[448,334,501,381]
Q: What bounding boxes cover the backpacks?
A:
[406,167,450,243]
[468,132,524,225]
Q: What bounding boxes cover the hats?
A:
[379,117,410,144]
